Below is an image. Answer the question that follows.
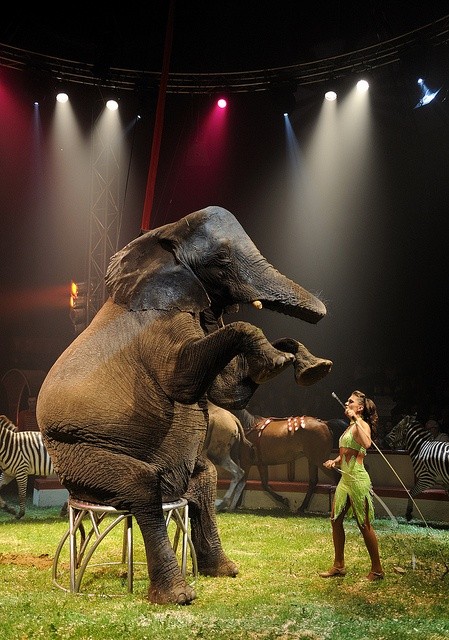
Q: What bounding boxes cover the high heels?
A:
[358,571,385,581]
[319,567,346,577]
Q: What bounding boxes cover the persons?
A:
[17,395,40,432]
[318,390,387,582]
[376,403,449,450]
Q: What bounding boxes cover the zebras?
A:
[384,412,449,521]
[0,415,54,519]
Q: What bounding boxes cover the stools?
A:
[52,497,198,598]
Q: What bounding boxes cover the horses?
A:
[224,408,349,513]
[202,400,257,513]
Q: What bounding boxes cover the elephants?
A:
[36,206,333,605]
